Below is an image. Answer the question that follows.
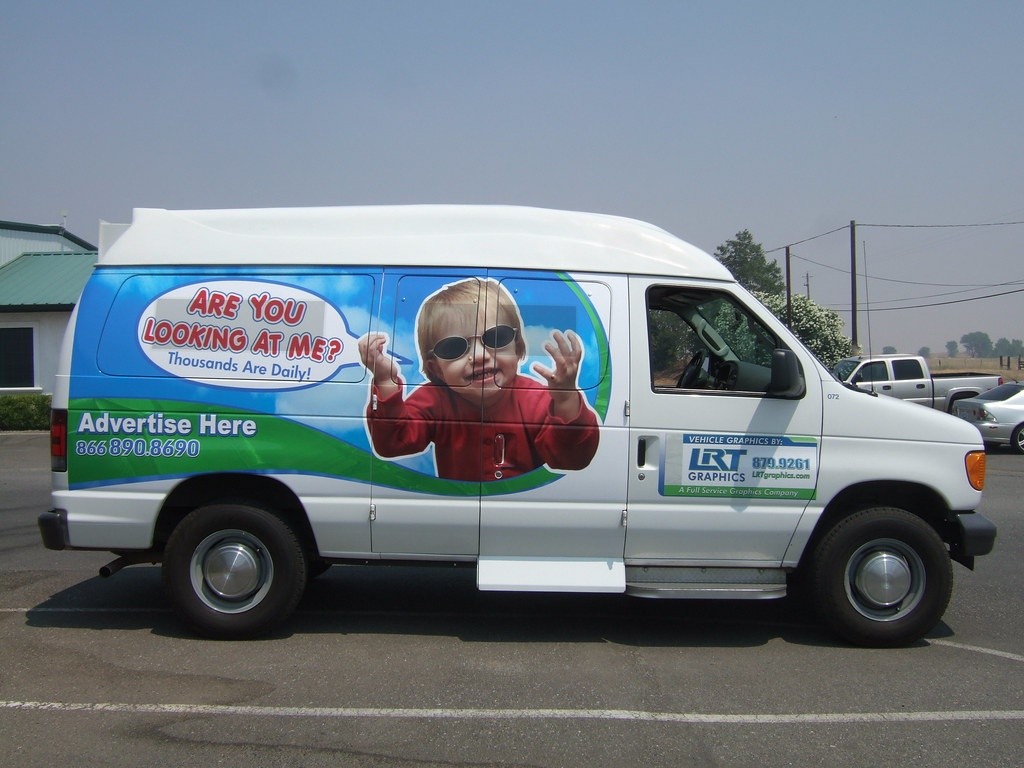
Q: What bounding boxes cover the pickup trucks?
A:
[826,353,1004,414]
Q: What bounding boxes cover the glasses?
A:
[427,324,518,361]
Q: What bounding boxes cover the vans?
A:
[35,205,998,647]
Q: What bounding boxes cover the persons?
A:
[357,275,601,481]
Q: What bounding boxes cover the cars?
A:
[951,379,1024,454]
